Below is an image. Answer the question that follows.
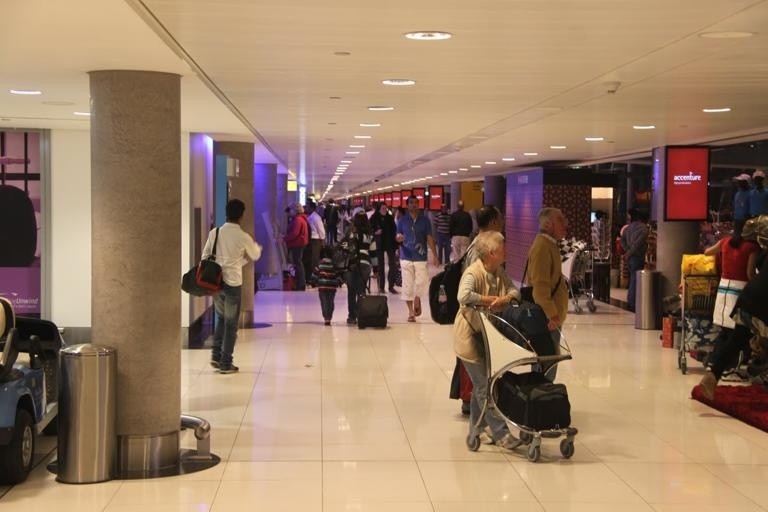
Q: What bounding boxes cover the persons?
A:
[732,173,752,234]
[524,206,570,381]
[453,205,505,415]
[750,170,768,216]
[620,210,647,310]
[275,192,505,326]
[704,218,759,372]
[452,228,524,449]
[200,198,263,374]
[700,249,768,403]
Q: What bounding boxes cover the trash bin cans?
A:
[635,270,662,330]
[56,342,118,484]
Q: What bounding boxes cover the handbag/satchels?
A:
[181,260,223,298]
[495,370,571,431]
[520,285,536,306]
[333,236,358,272]
[429,261,462,325]
[503,298,547,336]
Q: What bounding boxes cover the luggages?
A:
[355,276,388,328]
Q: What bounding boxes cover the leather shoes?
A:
[408,298,421,322]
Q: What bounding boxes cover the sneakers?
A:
[698,372,717,403]
[494,433,524,449]
[479,430,493,444]
[209,358,239,373]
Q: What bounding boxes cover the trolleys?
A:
[676,271,744,378]
[462,297,579,464]
[560,245,599,316]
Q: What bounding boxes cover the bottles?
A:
[438,285,448,314]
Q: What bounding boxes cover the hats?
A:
[732,171,753,186]
[288,201,302,211]
[751,170,766,180]
[353,207,366,218]
